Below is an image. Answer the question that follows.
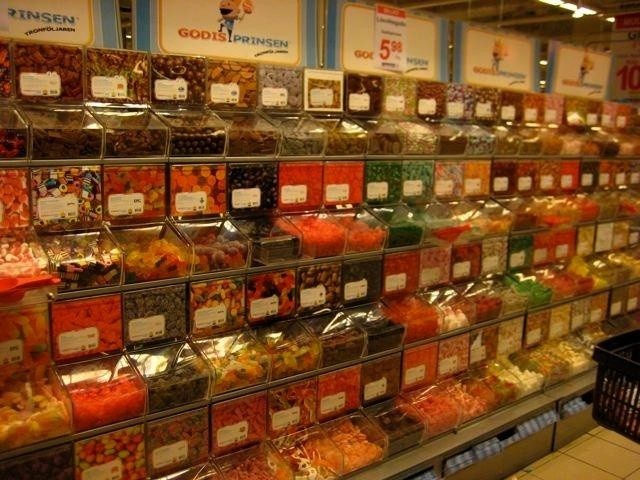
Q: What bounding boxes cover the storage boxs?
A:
[0,36,640,480]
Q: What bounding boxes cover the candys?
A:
[0,42,640,480]
